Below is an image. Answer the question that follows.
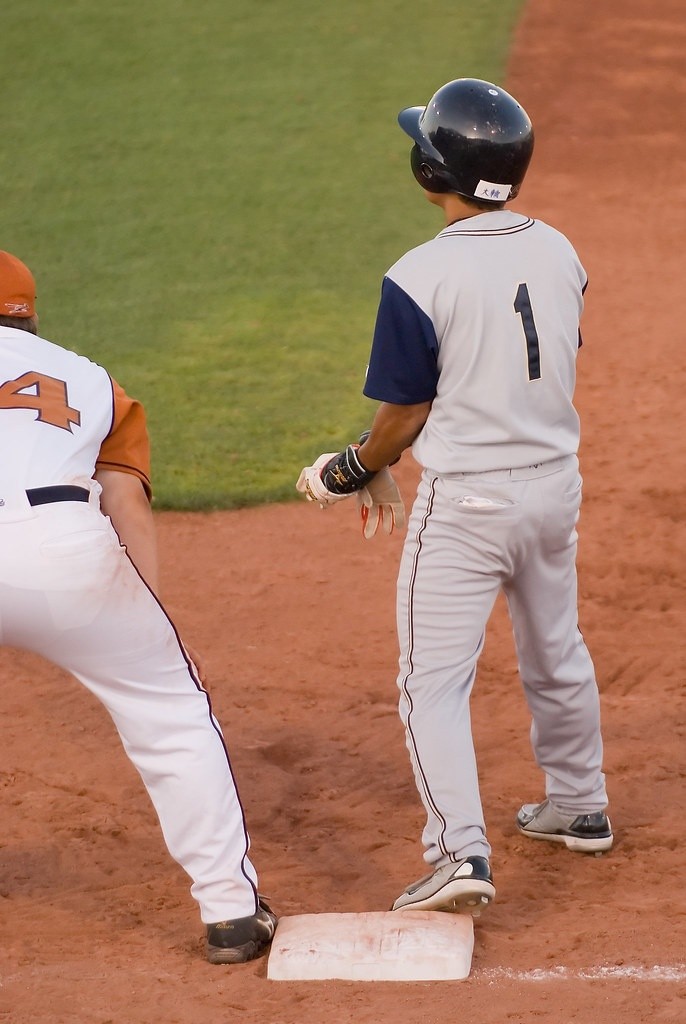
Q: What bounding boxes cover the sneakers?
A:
[388,855,497,919]
[513,799,615,852]
[202,891,279,965]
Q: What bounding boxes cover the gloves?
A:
[355,463,406,541]
[295,443,374,509]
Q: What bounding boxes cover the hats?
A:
[0,249,36,319]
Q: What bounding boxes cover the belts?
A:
[26,485,104,510]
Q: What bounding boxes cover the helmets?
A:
[398,74,536,205]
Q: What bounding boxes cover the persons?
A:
[0,250,278,965]
[295,78,613,918]
